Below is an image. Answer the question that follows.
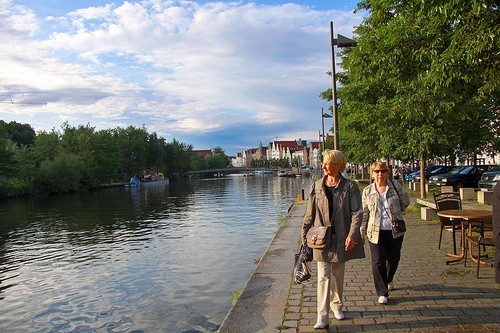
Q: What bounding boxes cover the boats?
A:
[244,173,255,177]
[125,176,140,187]
[277,170,288,177]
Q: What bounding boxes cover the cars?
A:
[408,165,435,181]
[405,166,420,181]
[477,165,500,190]
[428,165,499,191]
[423,165,458,180]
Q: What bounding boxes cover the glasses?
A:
[321,162,337,166]
[373,169,388,173]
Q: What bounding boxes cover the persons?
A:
[360,162,410,304]
[301,148,366,329]
[392,165,410,183]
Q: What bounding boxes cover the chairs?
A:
[432,190,487,255]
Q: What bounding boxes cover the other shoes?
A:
[332,310,345,320]
[388,281,395,291]
[313,322,330,329]
[378,296,388,303]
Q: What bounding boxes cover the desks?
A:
[436,209,494,267]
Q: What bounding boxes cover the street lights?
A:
[321,107,332,180]
[330,21,358,150]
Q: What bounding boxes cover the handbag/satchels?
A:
[305,225,332,249]
[300,242,313,262]
[293,243,312,285]
[391,219,406,233]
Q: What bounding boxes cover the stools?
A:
[463,228,496,279]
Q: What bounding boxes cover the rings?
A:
[351,244,354,247]
